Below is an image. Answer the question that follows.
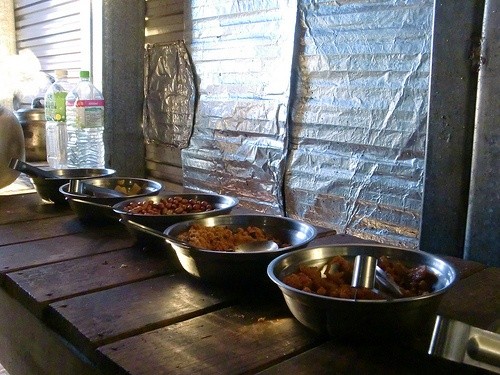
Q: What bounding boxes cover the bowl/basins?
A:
[163,213,318,280]
[58,178,163,225]
[28,167,116,204]
[113,193,239,241]
[267,242,458,339]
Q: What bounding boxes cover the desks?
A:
[0,189,500,375]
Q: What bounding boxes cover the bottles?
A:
[65,71,105,170]
[45,69,79,169]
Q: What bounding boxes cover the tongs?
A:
[67,179,128,198]
[427,313,500,375]
[8,158,64,179]
[350,254,406,300]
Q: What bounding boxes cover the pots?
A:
[14,96,48,160]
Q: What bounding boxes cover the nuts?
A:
[123,196,212,215]
